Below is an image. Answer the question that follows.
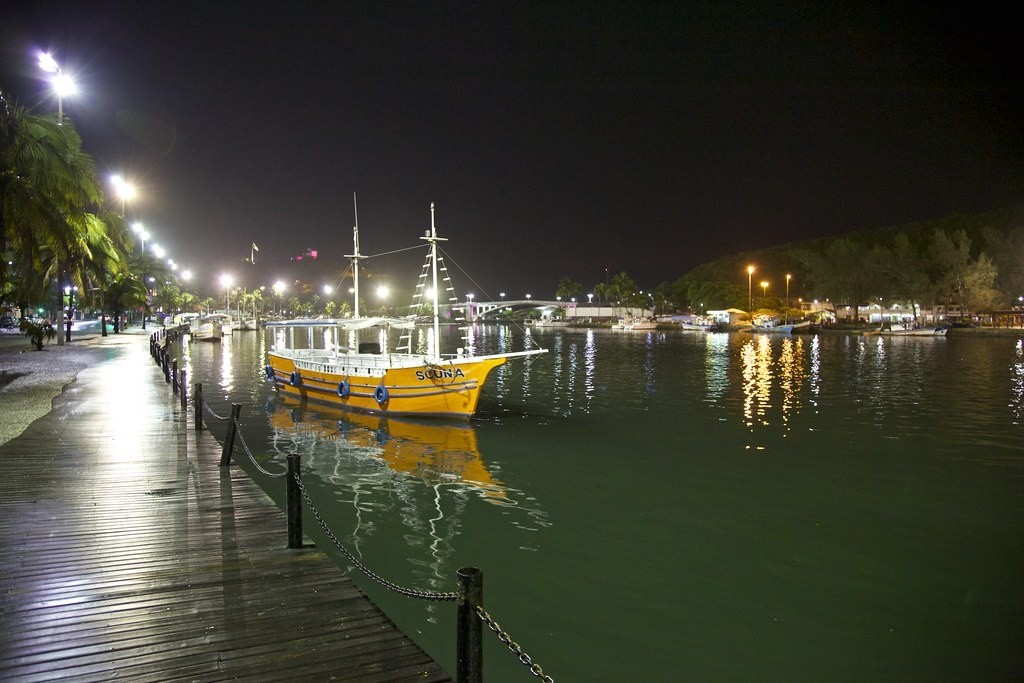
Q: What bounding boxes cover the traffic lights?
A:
[38,307,43,316]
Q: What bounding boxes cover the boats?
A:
[862,324,948,335]
[611,317,658,330]
[682,320,723,330]
[232,318,246,329]
[265,190,549,416]
[190,310,224,343]
[199,304,233,334]
[245,313,262,330]
[750,320,816,333]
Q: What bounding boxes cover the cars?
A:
[0,316,18,328]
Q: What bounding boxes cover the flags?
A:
[252,241,259,252]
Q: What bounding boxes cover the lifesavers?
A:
[374,385,388,404]
[266,365,273,379]
[289,372,299,386]
[337,379,350,398]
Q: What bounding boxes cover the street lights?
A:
[221,276,232,315]
[557,297,561,303]
[112,173,133,219]
[132,222,150,255]
[526,295,531,299]
[786,275,791,321]
[571,298,576,302]
[500,293,505,300]
[274,283,286,312]
[588,293,593,303]
[53,74,70,121]
[761,281,768,297]
[747,266,755,311]
[466,293,473,302]
